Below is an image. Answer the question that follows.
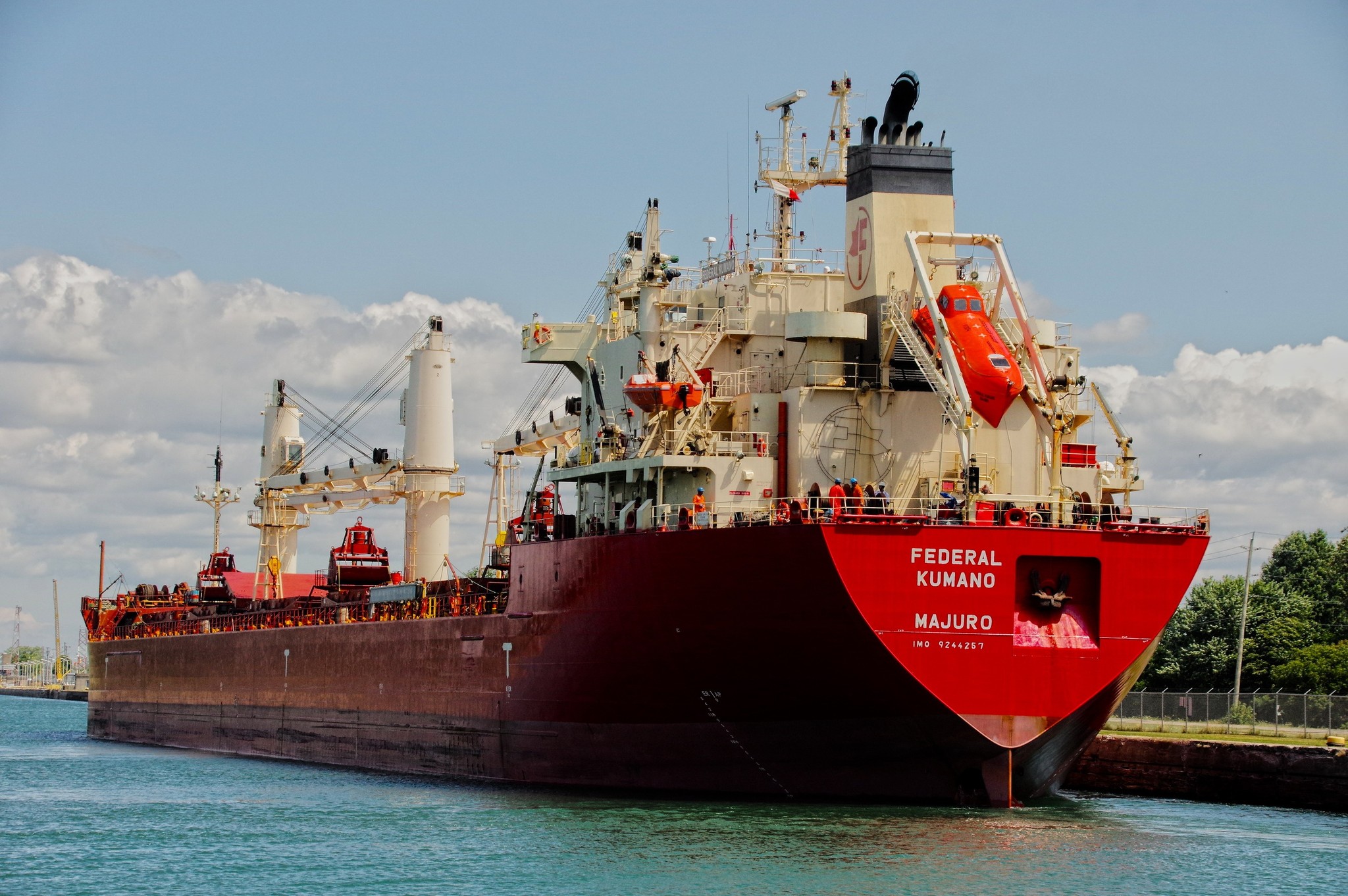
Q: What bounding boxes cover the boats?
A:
[913,285,1025,428]
[622,375,703,413]
[81,69,1213,811]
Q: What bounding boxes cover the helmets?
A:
[835,478,841,482]
[697,487,704,492]
[879,482,885,486]
[850,478,857,483]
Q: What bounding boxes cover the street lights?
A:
[7,656,72,686]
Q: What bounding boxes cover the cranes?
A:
[51,576,70,681]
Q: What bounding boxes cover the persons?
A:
[693,487,706,529]
[850,478,864,522]
[875,482,890,506]
[828,478,847,523]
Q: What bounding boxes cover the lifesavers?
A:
[970,271,979,279]
[756,437,767,457]
[514,431,521,446]
[776,502,790,523]
[322,496,327,502]
[533,327,551,345]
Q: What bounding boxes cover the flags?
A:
[770,178,799,200]
